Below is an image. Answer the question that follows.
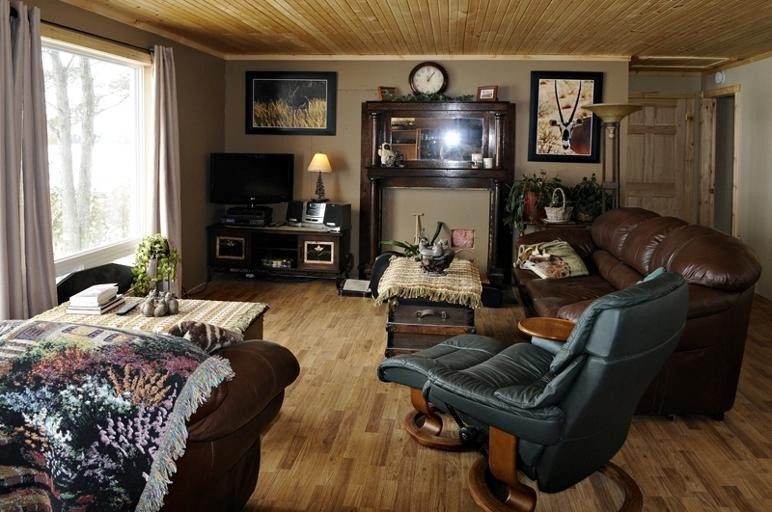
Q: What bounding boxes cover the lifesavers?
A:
[225,209,272,227]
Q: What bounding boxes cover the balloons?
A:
[408,62,448,97]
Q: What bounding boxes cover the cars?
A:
[471,153,493,170]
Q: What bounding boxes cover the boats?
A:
[286,200,303,223]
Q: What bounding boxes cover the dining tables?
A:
[127,233,183,317]
[503,170,613,234]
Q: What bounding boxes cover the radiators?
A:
[115,302,138,316]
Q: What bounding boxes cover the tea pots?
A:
[384,111,489,169]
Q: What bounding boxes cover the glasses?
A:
[580,103,643,216]
[307,153,333,203]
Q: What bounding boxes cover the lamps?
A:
[377,268,691,511]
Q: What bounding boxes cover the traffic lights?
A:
[514,239,589,279]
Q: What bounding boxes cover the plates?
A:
[205,223,355,295]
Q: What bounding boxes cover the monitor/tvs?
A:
[66,282,127,316]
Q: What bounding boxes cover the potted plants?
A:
[476,85,498,102]
[245,70,337,136]
[527,70,603,164]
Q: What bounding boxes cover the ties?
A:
[209,153,294,210]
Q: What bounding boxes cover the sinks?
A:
[0,317,301,511]
[511,207,761,421]
